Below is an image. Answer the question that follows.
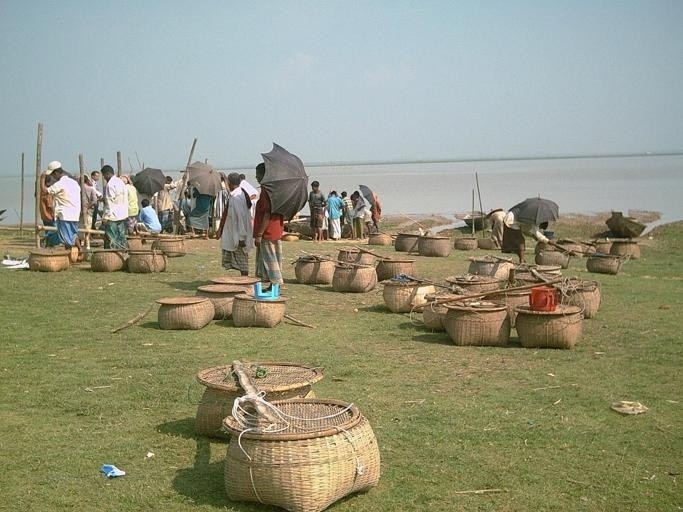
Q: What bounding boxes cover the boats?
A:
[603,210,648,238]
[462,208,492,232]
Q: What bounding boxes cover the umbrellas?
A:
[262,142,309,221]
[509,193,560,226]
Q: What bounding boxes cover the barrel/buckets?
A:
[532,287,557,311]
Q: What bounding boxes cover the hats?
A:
[45,161,61,175]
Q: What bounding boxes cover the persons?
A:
[308,181,382,242]
[256,163,284,295]
[34,160,258,277]
[486,208,506,248]
[501,212,554,266]
